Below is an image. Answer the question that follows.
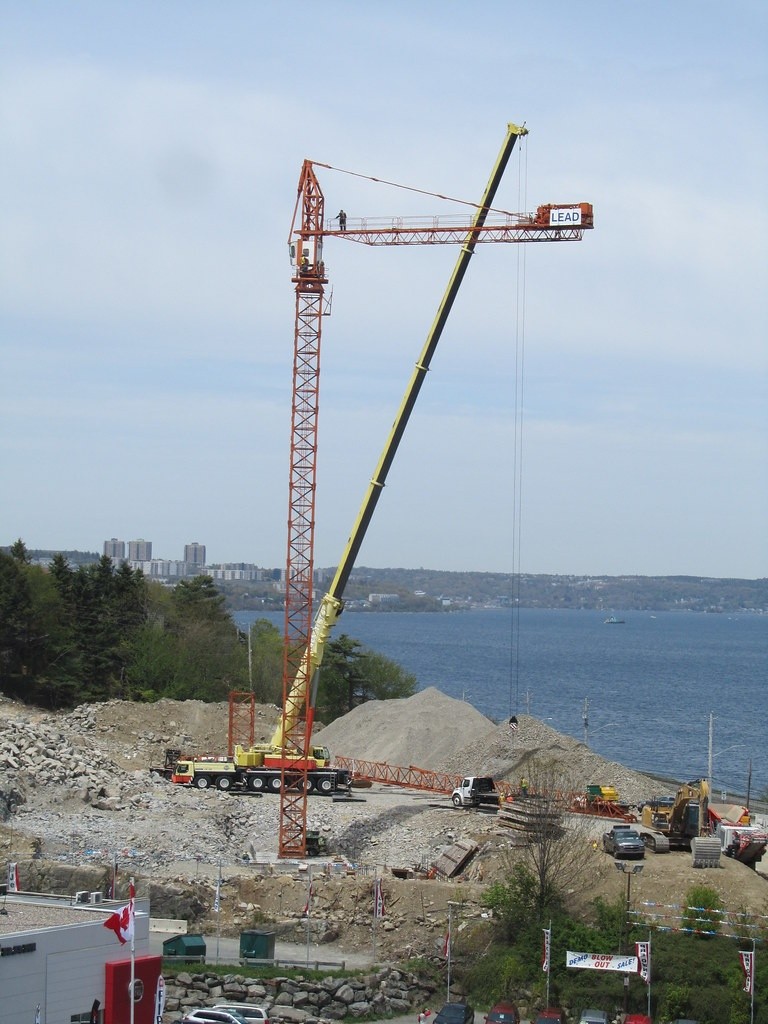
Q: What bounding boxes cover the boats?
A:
[603,615,627,623]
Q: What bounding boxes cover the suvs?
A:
[182,1006,270,1024]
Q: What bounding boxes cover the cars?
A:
[530,1008,566,1024]
[432,1004,475,1024]
[484,1004,520,1024]
[579,1009,651,1024]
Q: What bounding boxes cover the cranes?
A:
[276,157,598,861]
[228,692,638,823]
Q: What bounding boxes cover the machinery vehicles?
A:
[150,119,525,793]
[640,779,768,868]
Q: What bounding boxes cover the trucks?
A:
[451,776,563,807]
[574,785,637,815]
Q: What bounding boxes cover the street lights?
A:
[703,711,746,802]
[579,695,620,747]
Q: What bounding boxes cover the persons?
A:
[498,793,505,802]
[418,1009,431,1024]
[506,793,513,801]
[519,777,529,798]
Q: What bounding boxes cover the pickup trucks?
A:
[603,825,645,860]
[646,796,675,807]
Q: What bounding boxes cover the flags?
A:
[103,883,136,945]
